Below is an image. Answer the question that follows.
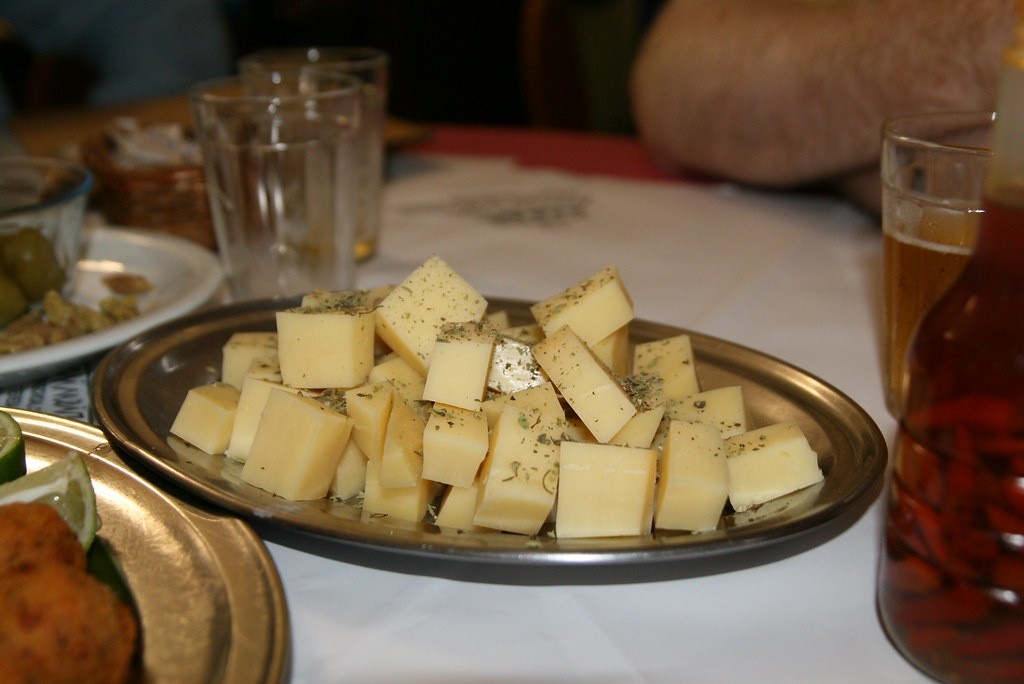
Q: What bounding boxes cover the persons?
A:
[628,0,1023,223]
[0,0,231,108]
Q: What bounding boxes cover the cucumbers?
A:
[0,411,26,486]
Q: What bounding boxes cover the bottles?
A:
[875,32,1024,683]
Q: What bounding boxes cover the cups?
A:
[194,69,371,301]
[877,111,996,421]
[230,43,391,265]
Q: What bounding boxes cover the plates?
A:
[0,407,291,684]
[0,206,223,387]
[83,115,400,235]
[92,293,888,588]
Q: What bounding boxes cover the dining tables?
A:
[1,94,937,684]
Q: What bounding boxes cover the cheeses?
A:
[171,247,824,540]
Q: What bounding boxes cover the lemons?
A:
[0,455,97,557]
